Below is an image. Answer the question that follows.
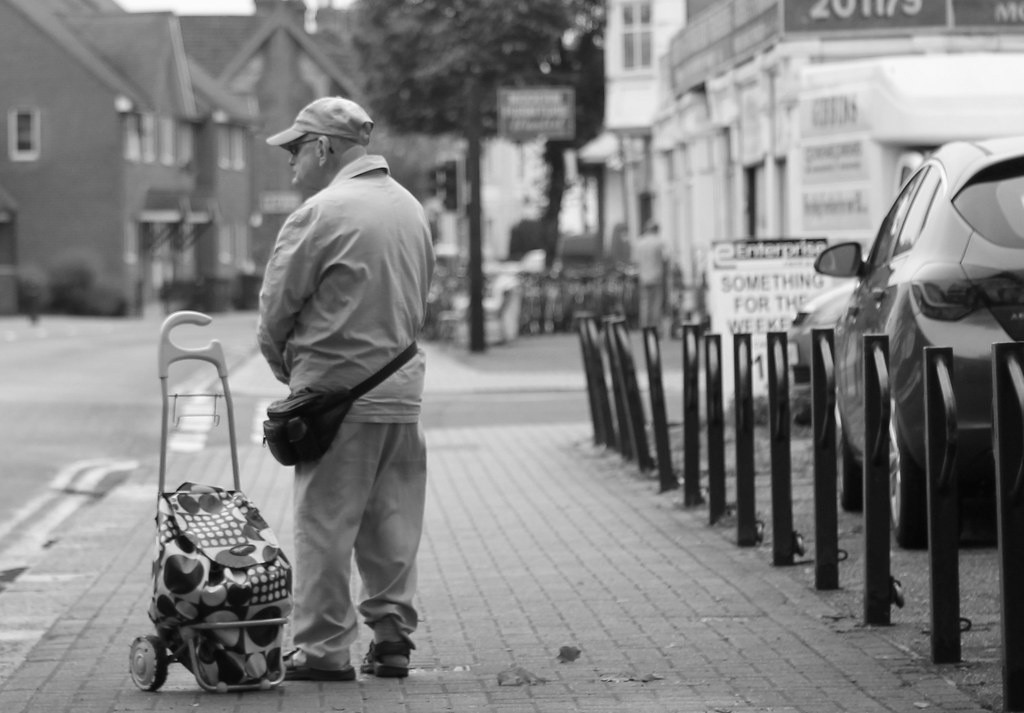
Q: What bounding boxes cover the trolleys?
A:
[130,311,289,693]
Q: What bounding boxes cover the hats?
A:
[267,95,376,147]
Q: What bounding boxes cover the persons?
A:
[258,94,436,680]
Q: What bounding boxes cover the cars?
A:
[787,280,862,389]
[812,132,1024,552]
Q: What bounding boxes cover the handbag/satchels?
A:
[264,341,420,467]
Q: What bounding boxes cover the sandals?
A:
[360,639,413,678]
[283,647,356,681]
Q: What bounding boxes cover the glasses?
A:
[289,137,336,157]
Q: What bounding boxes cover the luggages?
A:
[128,310,292,691]
